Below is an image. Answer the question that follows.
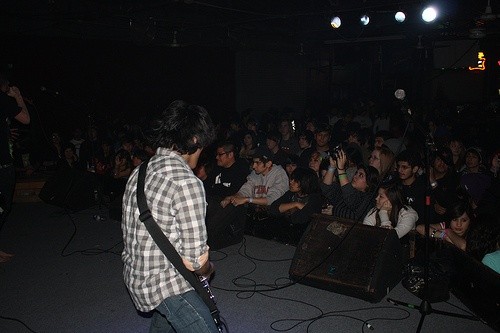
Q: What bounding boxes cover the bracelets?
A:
[444,228,453,234]
[249,197,253,203]
[337,172,348,179]
[440,232,446,240]
[432,229,437,238]
[327,165,336,172]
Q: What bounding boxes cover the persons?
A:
[167,87,499,275]
[121,101,221,333]
[0,62,29,263]
[7,114,159,203]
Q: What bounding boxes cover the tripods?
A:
[387,107,482,333]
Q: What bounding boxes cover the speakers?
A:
[206,201,249,250]
[288,214,404,304]
[450,256,500,332]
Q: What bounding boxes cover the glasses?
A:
[367,154,381,161]
[289,177,299,184]
[215,151,229,157]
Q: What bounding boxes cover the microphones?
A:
[395,89,417,119]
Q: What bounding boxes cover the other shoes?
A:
[40,195,64,208]
[0,247,16,264]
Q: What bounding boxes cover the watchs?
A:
[430,180,438,187]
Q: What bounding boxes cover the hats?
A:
[248,147,275,162]
[464,145,483,163]
[98,136,113,146]
[315,122,331,132]
[384,138,406,154]
